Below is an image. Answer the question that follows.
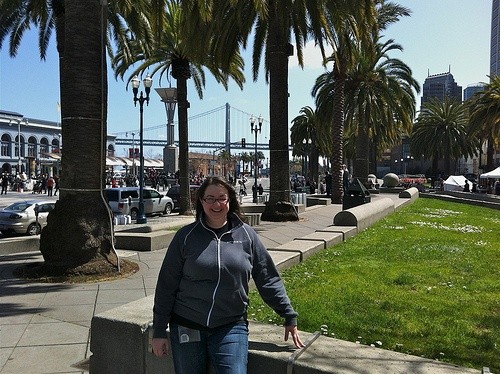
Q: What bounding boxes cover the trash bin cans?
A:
[342,178,371,210]
[310,185,315,194]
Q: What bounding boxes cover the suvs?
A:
[104,187,175,220]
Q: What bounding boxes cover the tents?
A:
[480,166,500,189]
[444,175,473,192]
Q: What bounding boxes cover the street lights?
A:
[126,132,141,178]
[249,113,264,203]
[8,117,29,174]
[131,72,153,224]
[302,138,312,179]
[406,156,414,166]
[394,159,403,175]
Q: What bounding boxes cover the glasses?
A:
[202,196,230,205]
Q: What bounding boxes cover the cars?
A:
[0,199,59,236]
[166,186,200,207]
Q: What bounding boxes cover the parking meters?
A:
[34,204,39,235]
[128,195,133,216]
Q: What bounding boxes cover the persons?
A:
[200,172,202,179]
[463,179,500,196]
[175,170,180,185]
[324,171,332,195]
[231,176,233,184]
[124,175,139,187]
[189,175,204,185]
[106,175,116,188]
[342,164,349,195]
[31,172,60,196]
[118,180,124,187]
[430,177,443,190]
[190,173,193,175]
[238,176,247,196]
[152,175,306,374]
[252,182,263,198]
[1,171,27,195]
[290,176,316,193]
[138,171,180,189]
